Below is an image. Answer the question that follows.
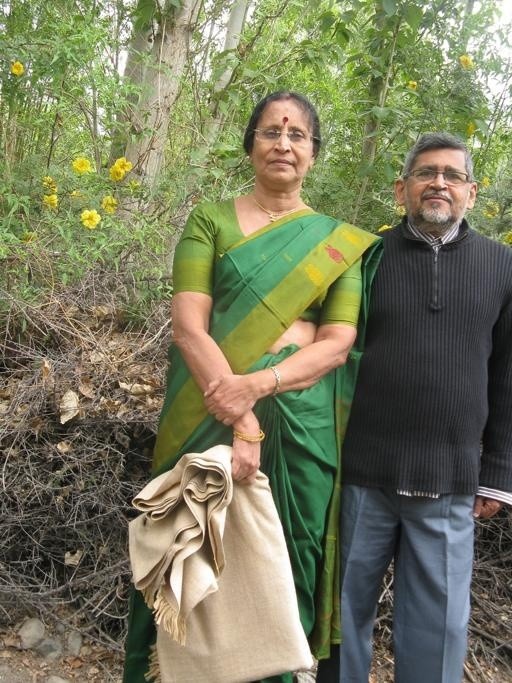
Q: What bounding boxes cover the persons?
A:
[329,131,511,682]
[122,85,382,682]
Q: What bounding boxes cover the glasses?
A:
[403,169,474,187]
[253,128,321,148]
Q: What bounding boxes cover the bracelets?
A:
[229,427,266,444]
[266,367,281,398]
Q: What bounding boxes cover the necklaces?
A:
[252,195,304,222]
